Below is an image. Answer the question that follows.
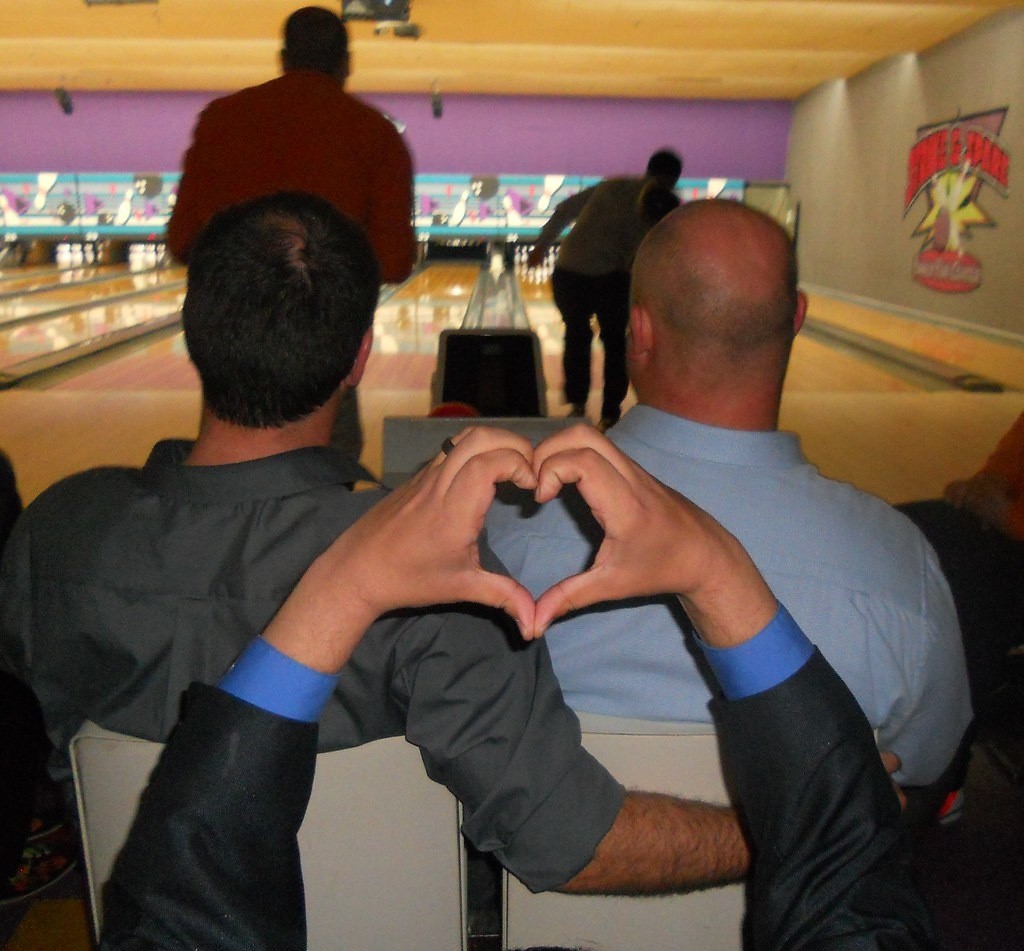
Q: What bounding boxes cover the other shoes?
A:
[601,405,623,426]
[568,403,586,418]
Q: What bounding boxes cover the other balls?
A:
[427,402,481,417]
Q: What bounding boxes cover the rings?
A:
[442,439,454,454]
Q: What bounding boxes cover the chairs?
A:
[71,718,469,951]
[502,714,744,951]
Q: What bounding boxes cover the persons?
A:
[0,194,905,895]
[488,199,975,786]
[0,454,79,904]
[942,407,1024,550]
[165,6,417,459]
[527,152,682,430]
[92,423,942,950]
[911,640,1024,951]
